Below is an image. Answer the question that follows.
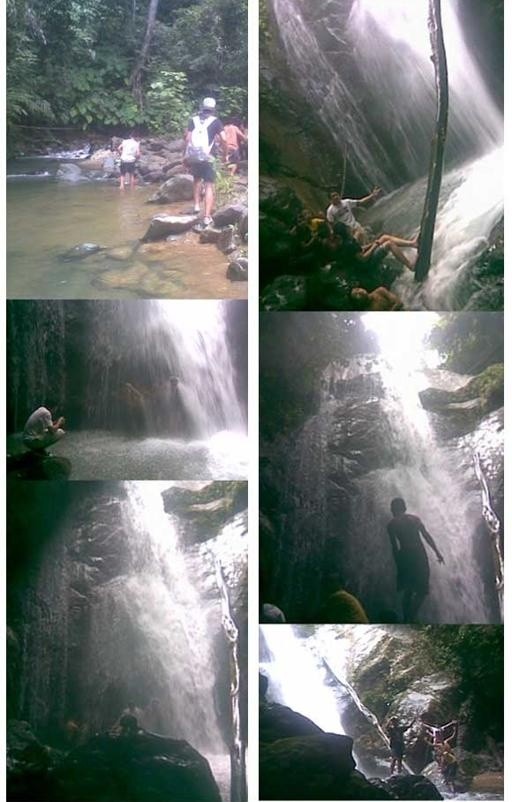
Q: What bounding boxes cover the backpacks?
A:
[186,116,217,161]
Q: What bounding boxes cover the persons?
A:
[260,604,286,622]
[384,715,417,778]
[117,129,141,191]
[183,97,248,226]
[319,573,371,623]
[387,498,447,624]
[422,721,459,797]
[287,184,421,271]
[111,700,177,738]
[349,284,405,310]
[23,398,65,457]
[380,607,400,623]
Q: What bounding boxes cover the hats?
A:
[202,97,216,108]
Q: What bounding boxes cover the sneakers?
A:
[193,205,221,228]
